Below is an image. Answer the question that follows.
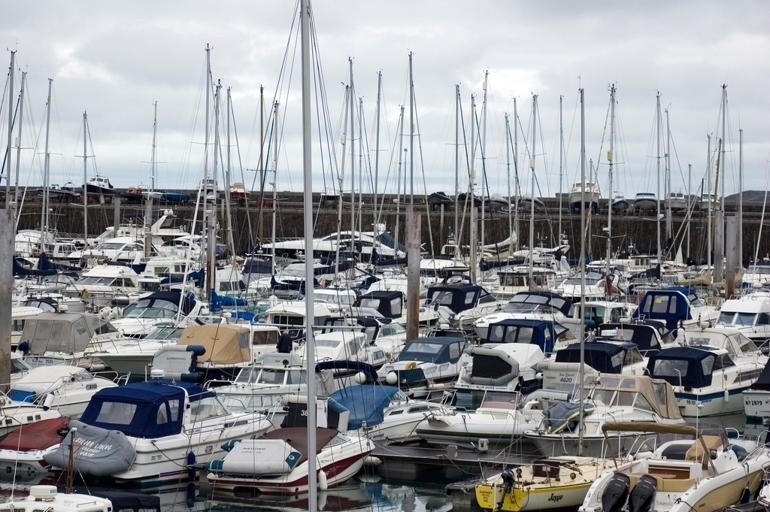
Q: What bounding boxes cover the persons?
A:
[276,329,292,353]
[18,338,30,354]
